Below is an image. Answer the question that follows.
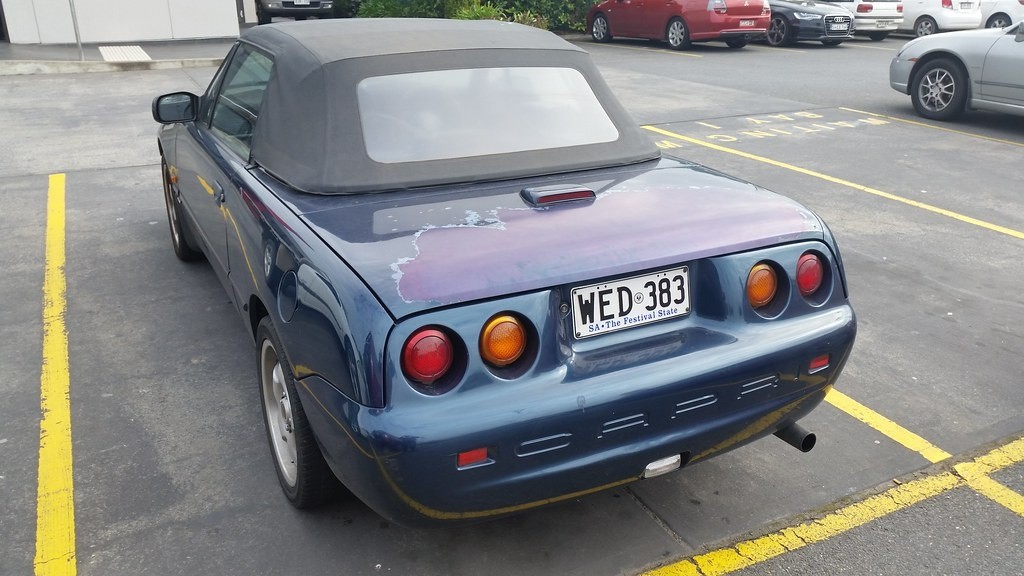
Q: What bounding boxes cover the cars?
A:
[745,0,855,46]
[818,0,904,41]
[900,0,983,37]
[254,0,340,26]
[585,0,771,51]
[889,20,1023,121]
[152,21,856,526]
